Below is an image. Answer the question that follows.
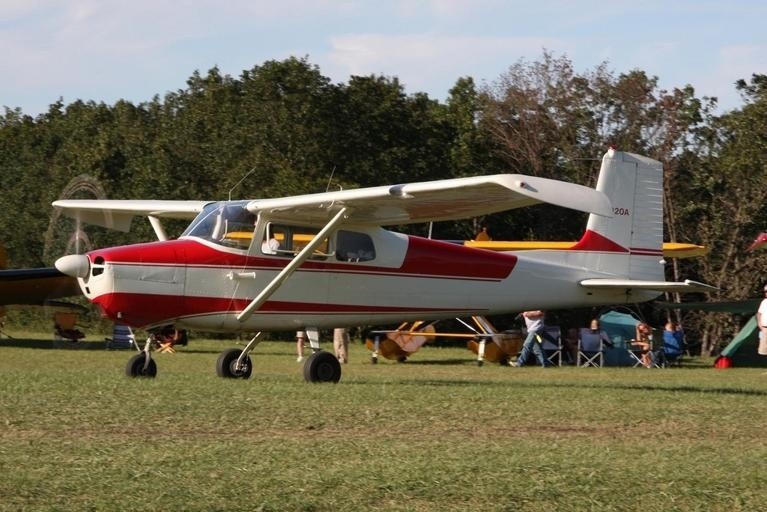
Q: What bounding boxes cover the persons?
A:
[757,285,767,355]
[296,331,306,362]
[262,224,281,255]
[657,322,684,367]
[508,310,551,368]
[333,328,349,364]
[580,319,614,348]
[630,322,653,368]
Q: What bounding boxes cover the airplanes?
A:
[0,147,720,383]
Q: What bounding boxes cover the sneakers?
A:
[509,361,521,367]
[296,356,303,361]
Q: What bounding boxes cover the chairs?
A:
[541,327,604,368]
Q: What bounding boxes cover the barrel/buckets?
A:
[113,323,134,345]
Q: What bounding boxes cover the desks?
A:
[625,348,661,369]
